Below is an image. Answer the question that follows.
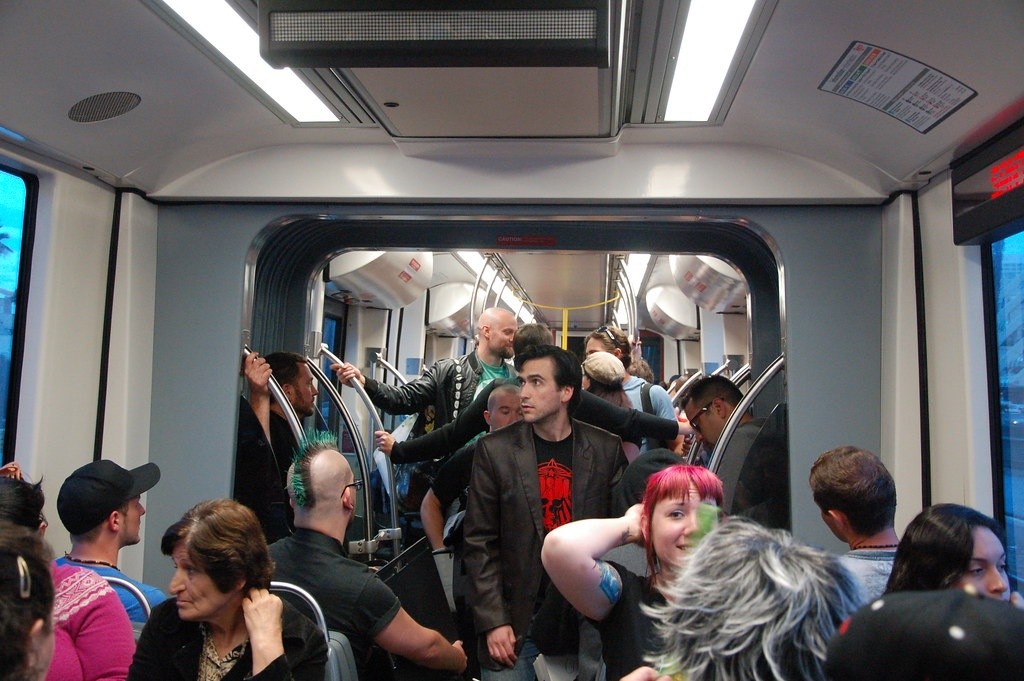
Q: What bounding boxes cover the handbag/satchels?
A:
[393,358,464,514]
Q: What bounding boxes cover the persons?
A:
[0,476,137,681]
[824,588,1024,681]
[540,464,725,681]
[471,343,631,681]
[126,498,330,681]
[266,432,468,681]
[885,503,1024,608]
[55,459,167,622]
[0,518,56,681]
[809,446,902,604]
[235,308,754,545]
[620,666,672,681]
[418,383,522,681]
[639,520,864,681]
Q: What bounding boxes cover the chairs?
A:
[99,575,359,681]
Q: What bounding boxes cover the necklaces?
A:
[850,544,899,549]
[63,550,122,570]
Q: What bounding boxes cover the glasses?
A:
[597,326,619,349]
[689,397,724,433]
[340,479,362,499]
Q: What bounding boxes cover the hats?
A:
[56,459,162,534]
[581,351,625,384]
[822,589,1024,681]
